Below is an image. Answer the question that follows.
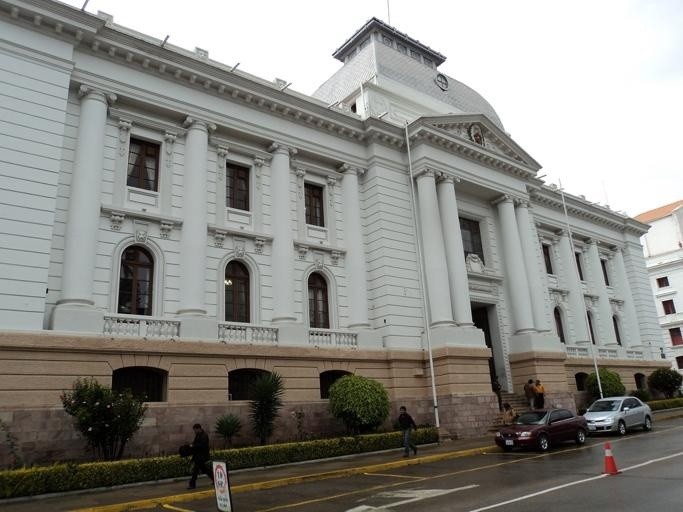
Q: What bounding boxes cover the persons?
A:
[523,379,539,410]
[491,375,503,410]
[398,406,418,457]
[502,403,516,426]
[185,423,213,489]
[533,379,545,408]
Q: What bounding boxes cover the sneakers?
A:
[414,448,417,454]
[186,486,195,489]
[403,455,408,457]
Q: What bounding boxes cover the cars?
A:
[494,408,588,451]
[581,395,653,436]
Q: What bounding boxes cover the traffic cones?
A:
[602,442,622,475]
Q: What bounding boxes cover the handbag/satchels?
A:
[179,445,192,456]
